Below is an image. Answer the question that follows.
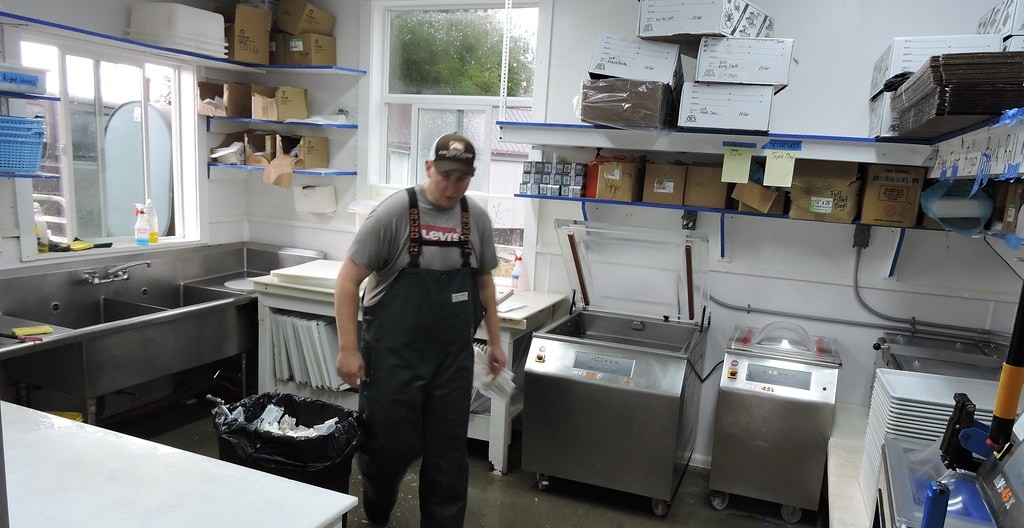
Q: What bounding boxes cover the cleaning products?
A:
[132,198,159,246]
[34,201,50,254]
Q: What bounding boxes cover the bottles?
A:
[512,251,522,293]
[146,199,159,245]
[33,202,49,253]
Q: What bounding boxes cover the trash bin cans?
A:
[219,391,359,528]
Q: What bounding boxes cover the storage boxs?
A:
[1002,182,1024,232]
[209,131,246,166]
[676,84,775,133]
[244,132,276,167]
[518,154,926,228]
[261,134,329,189]
[579,78,668,128]
[587,33,684,98]
[126,0,337,67]
[636,0,776,44]
[251,83,309,122]
[868,36,1003,140]
[694,36,794,94]
[197,81,250,119]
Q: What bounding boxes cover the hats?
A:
[428,131,475,178]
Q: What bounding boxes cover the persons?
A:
[335,130,506,528]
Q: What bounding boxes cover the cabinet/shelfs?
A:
[0,10,367,177]
[496,121,938,278]
[927,112,1024,282]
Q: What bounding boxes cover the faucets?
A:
[106,258,152,278]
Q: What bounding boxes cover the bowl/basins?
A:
[224,279,255,293]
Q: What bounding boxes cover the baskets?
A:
[0,114,45,174]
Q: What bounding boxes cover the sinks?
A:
[105,283,241,311]
[4,297,172,330]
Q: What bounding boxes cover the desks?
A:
[0,393,360,528]
[248,274,566,476]
